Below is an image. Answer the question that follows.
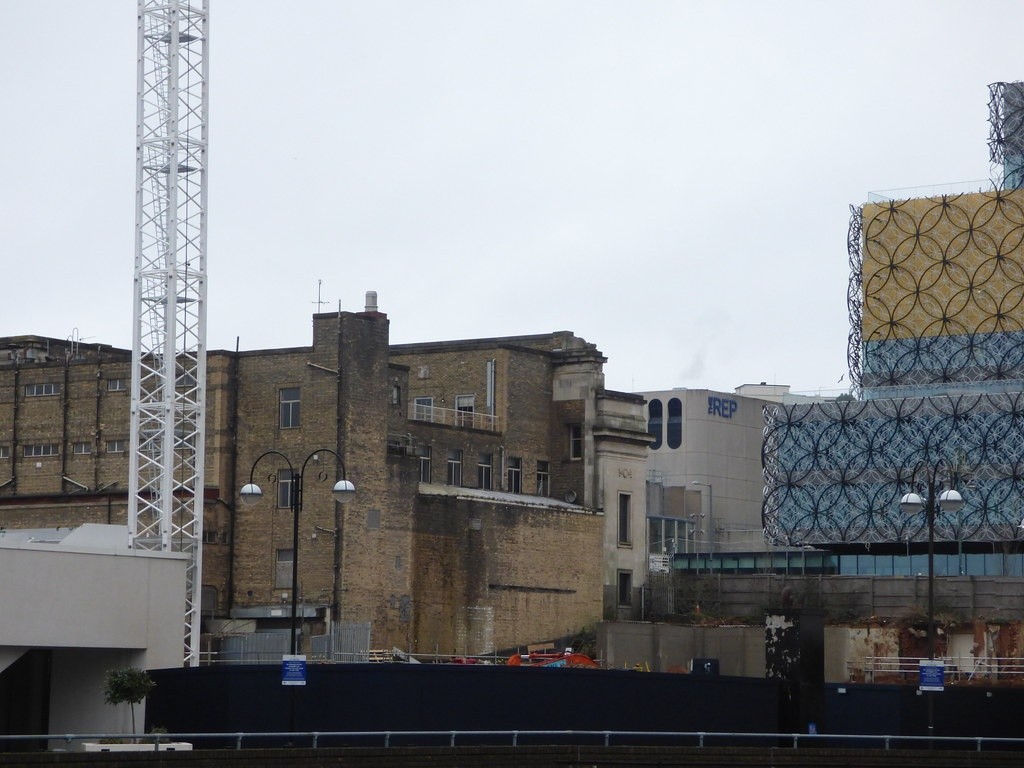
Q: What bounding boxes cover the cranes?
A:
[125,0,214,667]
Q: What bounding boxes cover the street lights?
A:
[900,459,965,749]
[239,449,357,655]
[692,482,713,577]
